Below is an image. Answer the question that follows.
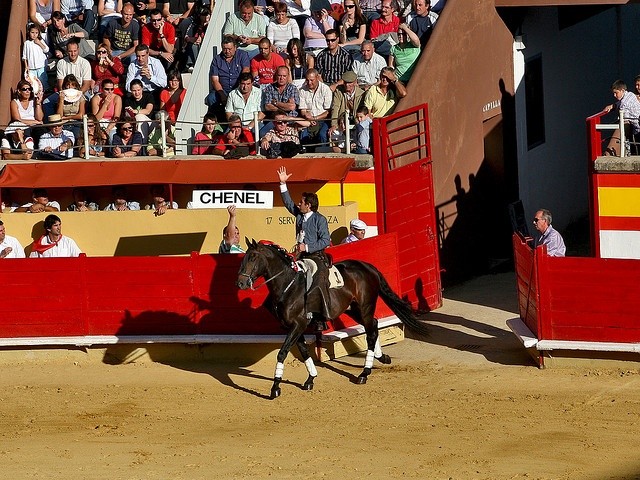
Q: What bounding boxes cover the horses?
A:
[236,236,430,397]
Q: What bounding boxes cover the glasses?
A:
[534,218,546,222]
[169,80,180,82]
[326,38,338,42]
[121,127,133,132]
[103,88,114,90]
[276,120,287,125]
[19,88,31,91]
[396,33,407,36]
[344,4,355,9]
[151,16,163,22]
[96,50,107,54]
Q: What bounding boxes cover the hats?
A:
[350,218,367,230]
[42,113,69,127]
[342,71,357,82]
[60,88,82,103]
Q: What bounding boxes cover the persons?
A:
[328,70,365,137]
[341,218,369,245]
[351,40,389,92]
[104,186,141,211]
[258,66,300,138]
[331,110,360,154]
[0,221,26,259]
[631,75,640,101]
[101,2,141,62]
[56,72,86,121]
[302,0,339,57]
[90,79,123,135]
[66,188,101,211]
[222,71,265,137]
[353,106,373,154]
[97,0,125,40]
[141,10,176,71]
[89,42,125,99]
[365,66,408,118]
[111,115,145,157]
[191,112,224,155]
[50,9,97,63]
[124,45,170,112]
[28,214,84,258]
[249,38,287,86]
[338,0,371,55]
[176,6,211,68]
[218,204,247,255]
[316,29,355,92]
[38,113,76,160]
[222,0,268,60]
[128,1,159,25]
[267,3,302,59]
[157,69,188,125]
[20,22,51,93]
[370,0,407,61]
[296,67,334,149]
[142,186,179,216]
[42,39,93,119]
[1,119,34,160]
[406,1,440,49]
[283,38,315,89]
[61,1,95,35]
[203,37,251,106]
[272,167,341,335]
[8,78,45,126]
[160,0,196,27]
[15,189,62,213]
[123,79,155,121]
[532,208,567,257]
[603,81,640,155]
[77,112,109,160]
[146,108,176,156]
[388,24,425,88]
[258,112,300,154]
[28,1,61,41]
[213,115,256,157]
